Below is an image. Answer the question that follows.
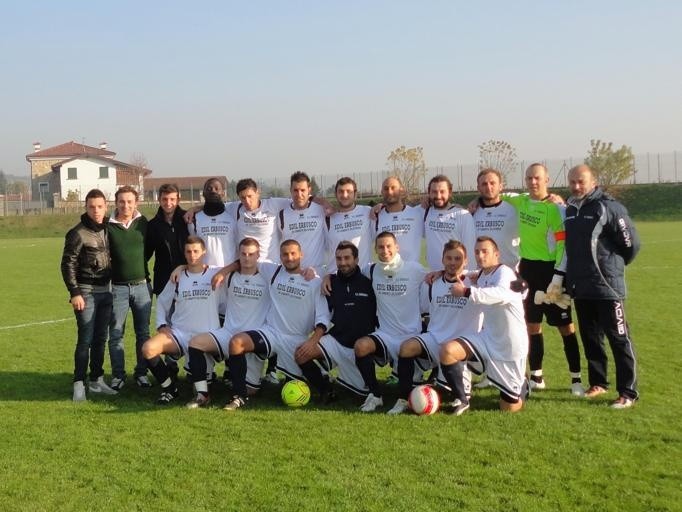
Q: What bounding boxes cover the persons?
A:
[466,162,585,398]
[145,182,190,386]
[419,165,566,391]
[181,178,336,385]
[320,230,427,414]
[167,237,318,412]
[140,234,227,405]
[421,236,530,414]
[277,170,328,284]
[105,183,155,391]
[294,240,378,405]
[324,177,375,278]
[384,239,530,417]
[367,174,478,273]
[563,163,642,409]
[186,179,236,388]
[61,188,121,404]
[208,239,332,411]
[371,176,464,390]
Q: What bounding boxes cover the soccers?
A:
[282,380,310,407]
[409,385,441,416]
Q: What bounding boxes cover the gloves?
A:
[533,273,572,311]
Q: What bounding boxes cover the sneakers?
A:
[155,386,182,405]
[222,393,249,411]
[448,399,471,417]
[72,380,87,402]
[360,395,385,413]
[88,376,120,395]
[385,398,410,416]
[184,392,213,410]
[381,375,399,387]
[584,384,608,398]
[135,374,153,391]
[110,376,128,391]
[473,376,493,389]
[264,370,281,385]
[609,395,636,409]
[570,382,585,397]
[529,379,546,390]
